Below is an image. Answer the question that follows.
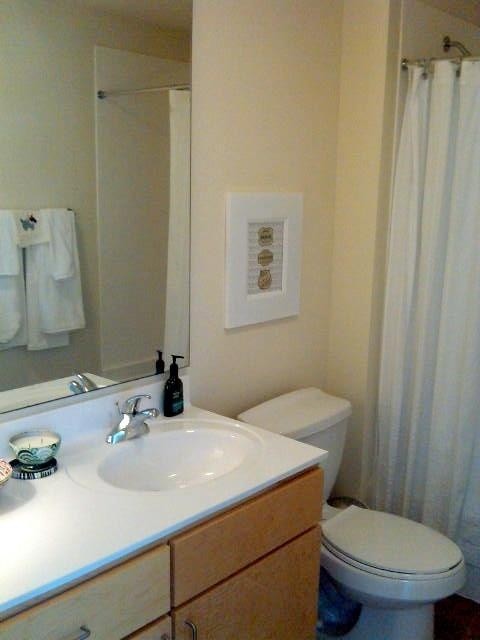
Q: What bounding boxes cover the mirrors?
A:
[0,2,193,417]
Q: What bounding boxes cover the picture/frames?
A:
[224,192,300,329]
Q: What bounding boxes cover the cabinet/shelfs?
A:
[1,466,325,640]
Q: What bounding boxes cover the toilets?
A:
[237,386,468,640]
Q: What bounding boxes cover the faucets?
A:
[105,394,161,444]
[67,374,97,394]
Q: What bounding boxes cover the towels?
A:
[0,206,87,352]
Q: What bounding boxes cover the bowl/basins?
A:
[7,429,64,472]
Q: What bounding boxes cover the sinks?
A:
[0,388,65,420]
[98,425,253,493]
